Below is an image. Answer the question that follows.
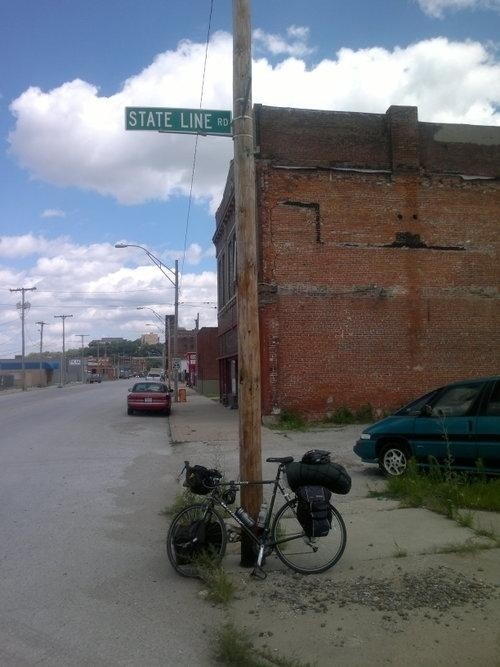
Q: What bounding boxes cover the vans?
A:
[146,370,163,381]
[351,374,500,478]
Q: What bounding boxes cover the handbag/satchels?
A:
[297,485,332,536]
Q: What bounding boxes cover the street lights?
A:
[136,304,171,370]
[144,323,165,334]
[113,236,181,402]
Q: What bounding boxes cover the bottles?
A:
[234,505,255,528]
[256,501,269,533]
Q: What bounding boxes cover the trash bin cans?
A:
[179,389,186,403]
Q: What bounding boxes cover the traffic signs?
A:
[124,105,233,135]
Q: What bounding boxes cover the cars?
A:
[120,369,147,379]
[125,381,175,416]
[90,372,102,382]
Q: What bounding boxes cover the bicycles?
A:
[164,454,350,581]
